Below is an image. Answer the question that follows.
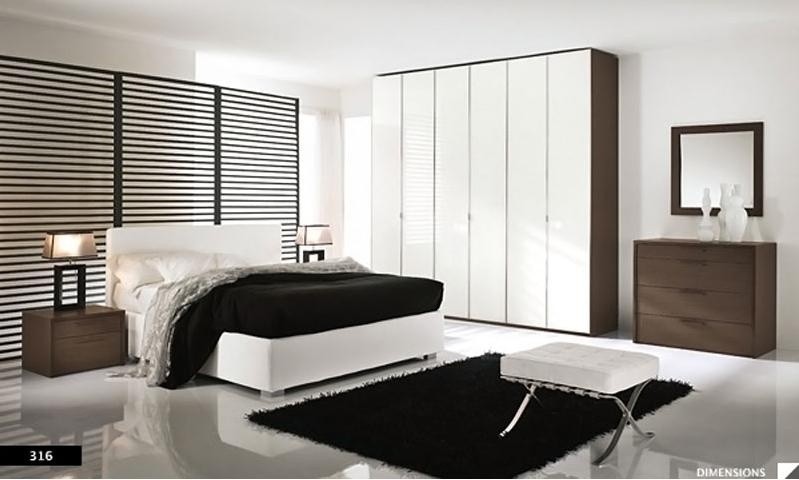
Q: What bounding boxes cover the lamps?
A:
[41,232,98,312]
[294,225,332,263]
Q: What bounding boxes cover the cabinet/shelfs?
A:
[633,237,777,359]
[372,48,620,338]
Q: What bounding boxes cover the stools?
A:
[499,341,660,465]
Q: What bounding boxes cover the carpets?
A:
[244,352,693,479]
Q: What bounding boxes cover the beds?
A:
[106,222,445,399]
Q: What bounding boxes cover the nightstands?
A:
[22,305,126,378]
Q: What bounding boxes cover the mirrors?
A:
[672,122,764,216]
